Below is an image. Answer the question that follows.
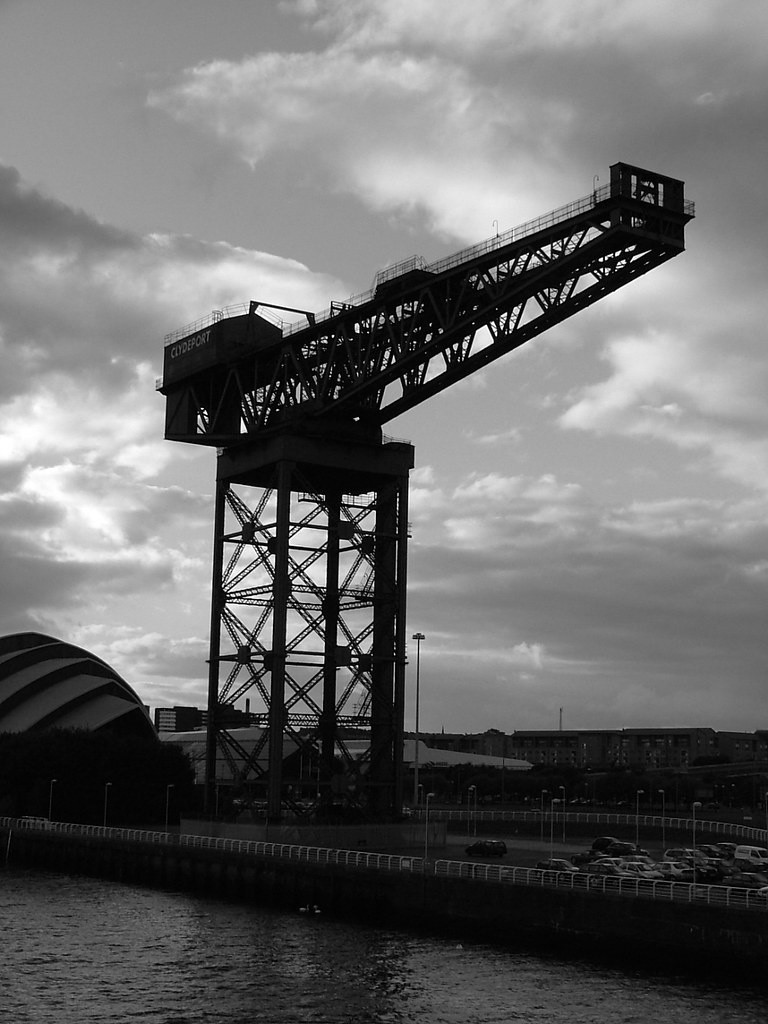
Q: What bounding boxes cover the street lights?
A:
[418,783,423,809]
[551,798,565,844]
[467,786,473,837]
[540,789,548,841]
[412,633,426,810]
[692,802,702,900]
[470,784,477,837]
[765,792,768,830]
[424,793,435,858]
[103,782,113,826]
[636,789,645,845]
[714,784,736,810]
[558,785,565,844]
[165,784,174,833]
[49,779,57,821]
[659,789,665,850]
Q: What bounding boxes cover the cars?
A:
[569,797,633,809]
[534,836,768,899]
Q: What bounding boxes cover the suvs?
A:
[465,839,507,858]
